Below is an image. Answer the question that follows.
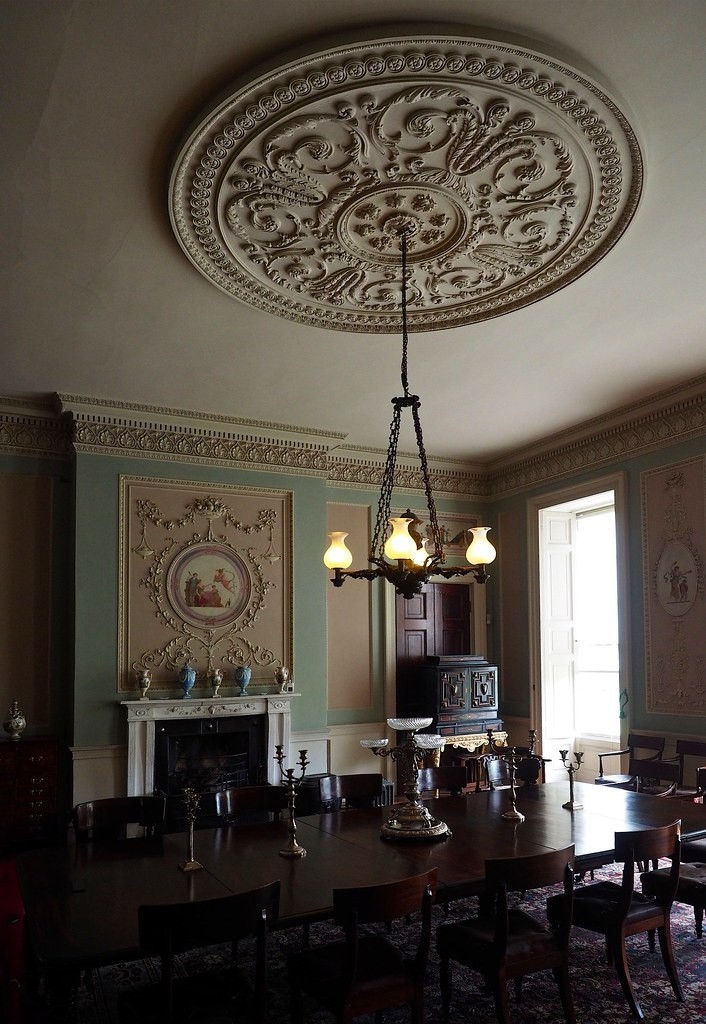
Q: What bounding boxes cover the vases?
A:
[274,666,290,693]
[177,662,196,698]
[235,666,252,696]
[134,670,153,701]
[3,700,26,739]
[207,669,223,697]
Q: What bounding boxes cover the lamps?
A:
[323,214,497,597]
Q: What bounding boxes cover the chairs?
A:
[0,734,706,1024]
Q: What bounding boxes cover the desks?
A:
[0,780,706,999]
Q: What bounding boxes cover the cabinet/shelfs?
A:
[411,654,503,735]
[121,695,296,798]
